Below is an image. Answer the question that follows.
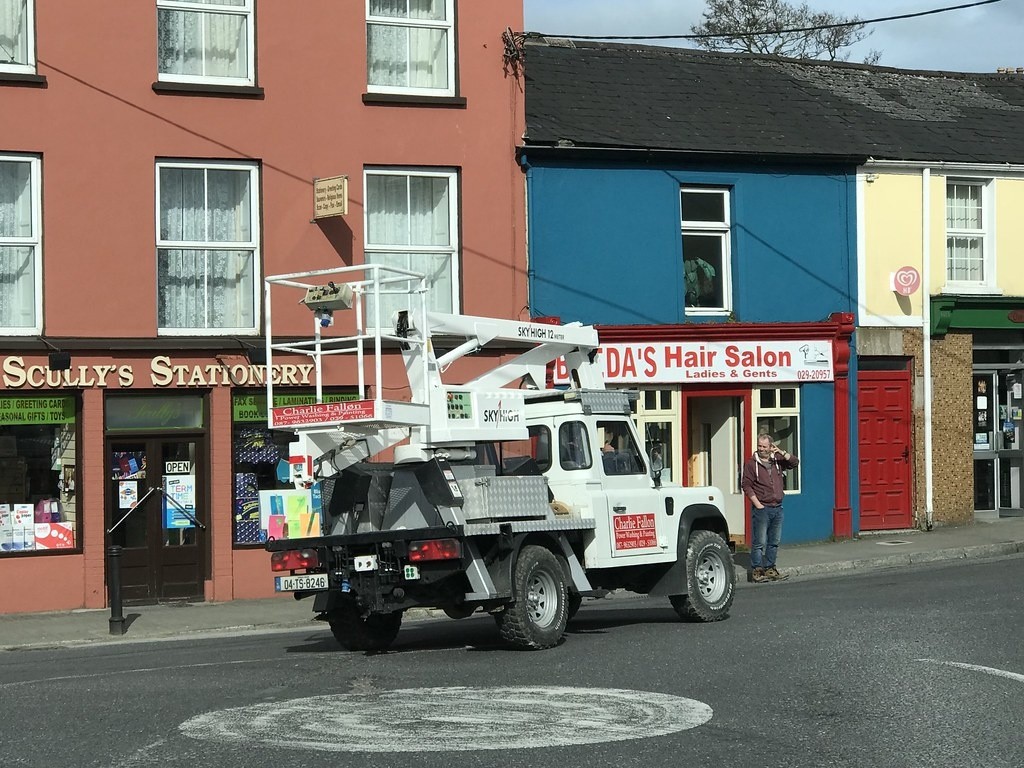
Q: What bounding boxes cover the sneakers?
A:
[764,568,789,582]
[751,568,770,583]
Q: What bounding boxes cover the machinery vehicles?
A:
[257,264,739,653]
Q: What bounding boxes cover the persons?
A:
[537,425,619,475]
[741,434,799,582]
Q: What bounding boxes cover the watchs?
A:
[782,449,786,457]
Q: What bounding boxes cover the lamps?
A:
[233,336,267,365]
[36,335,71,371]
